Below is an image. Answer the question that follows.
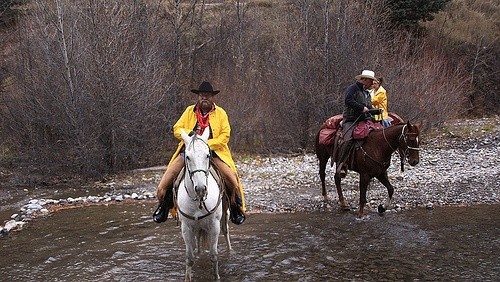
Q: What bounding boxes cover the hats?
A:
[378,77,383,84]
[355,69,377,80]
[191,81,220,95]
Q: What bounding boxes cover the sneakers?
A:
[338,164,347,177]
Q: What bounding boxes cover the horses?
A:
[314,120,423,219]
[176,126,234,282]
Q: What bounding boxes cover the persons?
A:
[337,70,388,176]
[152,82,246,225]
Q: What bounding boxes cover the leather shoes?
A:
[230,201,245,224]
[153,198,174,223]
